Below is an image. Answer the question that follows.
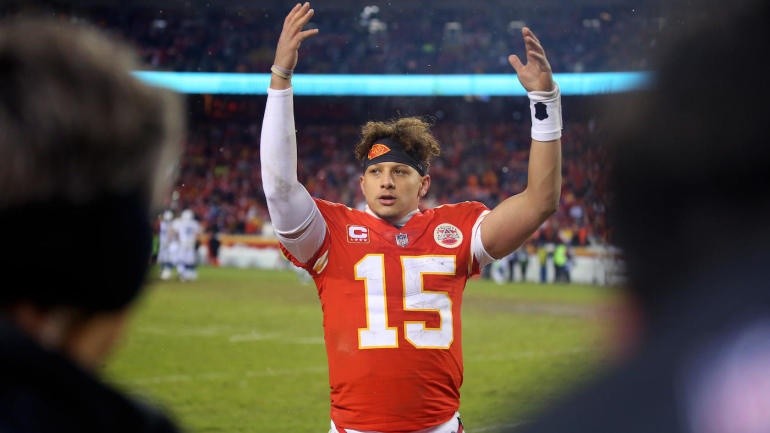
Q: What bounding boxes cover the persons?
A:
[170,207,203,283]
[0,0,652,285]
[156,209,174,281]
[536,243,549,285]
[506,1,770,432]
[0,13,187,433]
[553,242,571,285]
[260,1,564,432]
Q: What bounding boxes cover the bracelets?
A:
[270,63,295,79]
